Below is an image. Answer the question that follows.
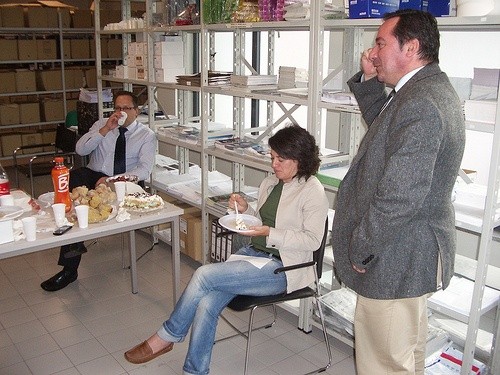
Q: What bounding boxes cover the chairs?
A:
[215,215,333,375]
[84,174,155,269]
[12,123,78,197]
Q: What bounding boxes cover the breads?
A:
[70,184,116,225]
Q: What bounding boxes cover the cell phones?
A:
[53,225,72,235]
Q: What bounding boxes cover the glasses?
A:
[113,107,135,111]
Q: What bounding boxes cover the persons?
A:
[332,10,464,374]
[41,91,158,292]
[124,125,329,375]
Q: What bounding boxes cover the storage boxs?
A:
[155,191,183,231]
[399,0,450,17]
[175,202,211,261]
[349,0,400,19]
[0,0,149,156]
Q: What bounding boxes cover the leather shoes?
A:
[64,245,86,258]
[40,269,78,291]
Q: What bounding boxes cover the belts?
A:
[249,245,282,260]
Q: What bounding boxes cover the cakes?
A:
[124,191,163,209]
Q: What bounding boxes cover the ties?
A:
[113,126,128,176]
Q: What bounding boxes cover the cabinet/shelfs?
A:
[94,0,500,375]
[0,0,146,167]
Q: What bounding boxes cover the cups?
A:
[22,217,37,242]
[75,205,89,228]
[1,195,13,206]
[117,111,127,126]
[51,203,66,228]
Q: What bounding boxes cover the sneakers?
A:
[124,340,174,364]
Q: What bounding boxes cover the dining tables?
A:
[0,198,184,311]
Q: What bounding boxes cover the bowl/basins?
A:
[9,190,29,206]
[105,174,138,184]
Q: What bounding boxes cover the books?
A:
[205,189,258,214]
[148,66,351,174]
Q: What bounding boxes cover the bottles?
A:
[51,157,72,213]
[0,161,10,196]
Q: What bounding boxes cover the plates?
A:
[218,214,263,233]
[38,192,55,205]
[105,204,117,221]
[120,196,164,213]
